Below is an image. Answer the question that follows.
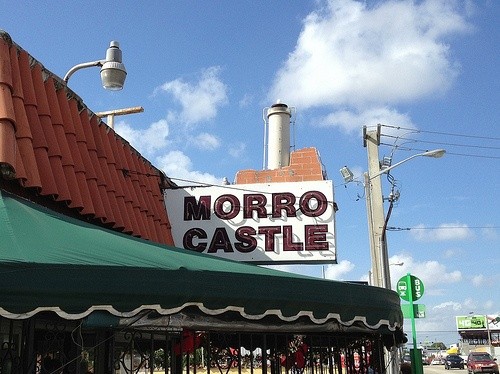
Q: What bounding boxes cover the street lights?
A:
[363,149,447,290]
[469,311,492,356]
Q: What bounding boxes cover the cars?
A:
[443,355,464,370]
[464,351,499,374]
[403,352,445,365]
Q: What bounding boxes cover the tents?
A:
[0,166,405,374]
